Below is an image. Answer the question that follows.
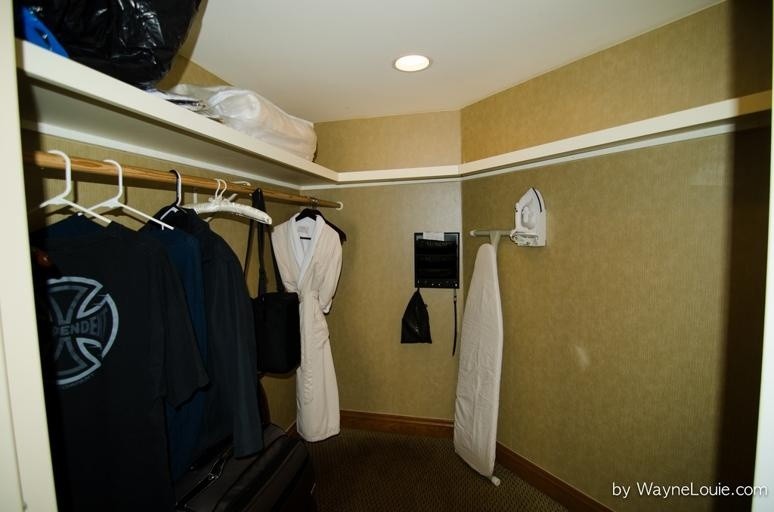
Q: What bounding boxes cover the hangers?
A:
[27,149,348,246]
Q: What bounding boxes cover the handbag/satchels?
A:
[168,424,316,511]
[401,290,432,345]
[252,289,302,377]
